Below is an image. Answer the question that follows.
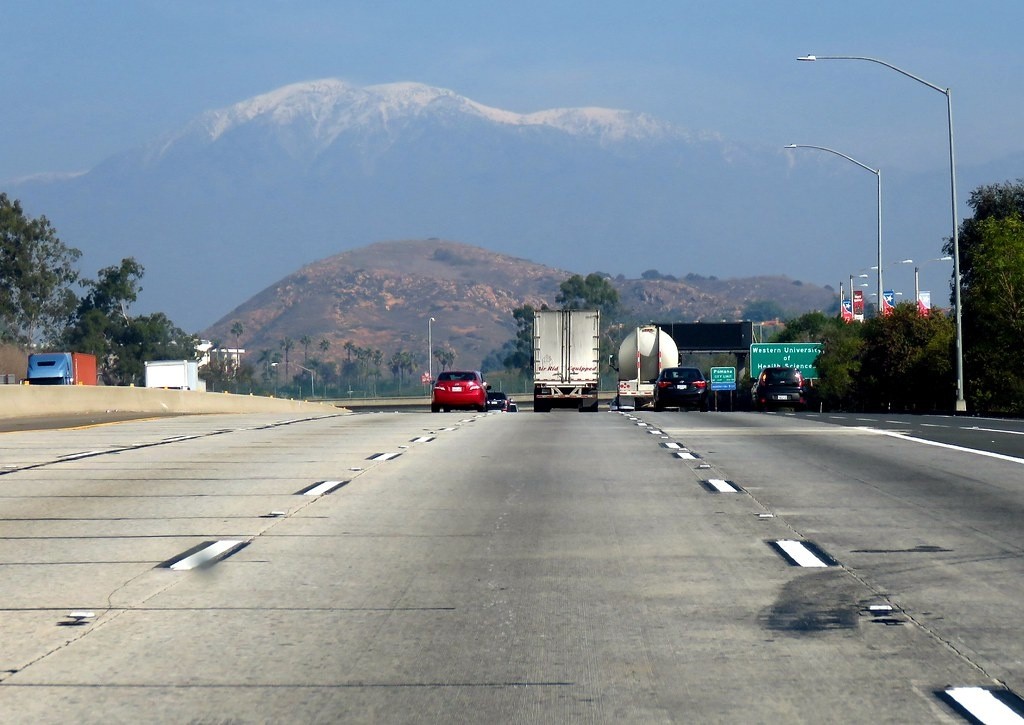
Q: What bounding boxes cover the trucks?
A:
[144,360,199,390]
[533,309,601,412]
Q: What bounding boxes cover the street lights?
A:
[915,256,954,304]
[428,317,436,395]
[849,266,878,321]
[783,144,884,313]
[271,362,314,396]
[798,54,968,414]
[839,258,913,319]
[759,322,764,343]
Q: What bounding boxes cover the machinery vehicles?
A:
[609,324,683,411]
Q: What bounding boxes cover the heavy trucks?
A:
[20,352,97,386]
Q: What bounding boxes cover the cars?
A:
[653,369,711,412]
[487,391,511,411]
[750,366,808,413]
[510,405,518,412]
[428,370,491,412]
[607,398,617,411]
